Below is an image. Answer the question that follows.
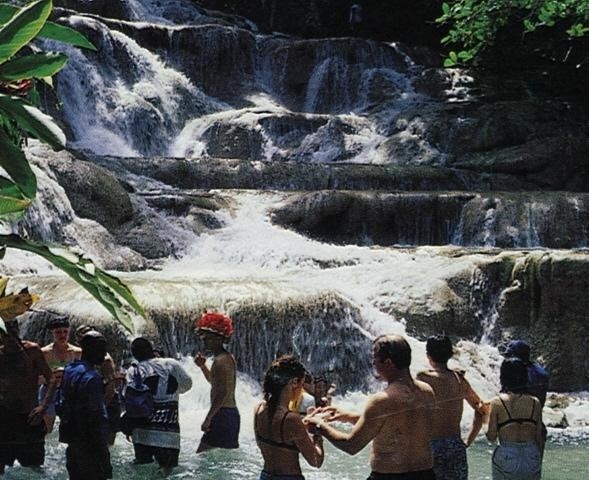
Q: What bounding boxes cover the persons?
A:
[303,334,437,480]
[0,316,192,480]
[417,334,484,480]
[505,338,549,408]
[193,314,240,453]
[250,356,323,480]
[485,358,544,480]
[288,369,331,416]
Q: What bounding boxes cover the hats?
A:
[46,317,71,328]
[194,315,233,338]
[0,319,18,330]
[500,340,529,358]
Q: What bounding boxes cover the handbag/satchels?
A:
[60,405,91,444]
[123,381,154,420]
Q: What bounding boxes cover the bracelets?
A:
[315,421,321,435]
